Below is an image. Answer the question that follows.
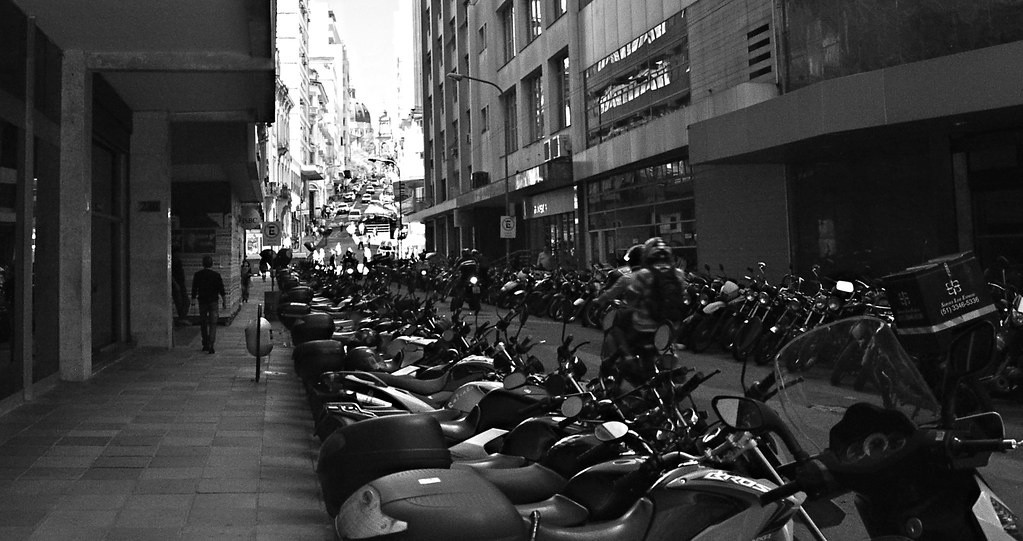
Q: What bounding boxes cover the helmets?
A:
[628,244,647,271]
[646,239,672,258]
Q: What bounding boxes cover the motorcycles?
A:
[276,247,1023,541]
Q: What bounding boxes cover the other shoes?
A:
[209,348,215,354]
[615,354,635,369]
[202,347,209,352]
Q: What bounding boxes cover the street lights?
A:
[445,72,511,270]
[367,157,403,259]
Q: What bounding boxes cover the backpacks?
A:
[647,264,685,323]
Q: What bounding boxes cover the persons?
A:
[625,236,688,365]
[258,171,580,313]
[240,259,253,303]
[189,255,228,355]
[589,245,655,381]
